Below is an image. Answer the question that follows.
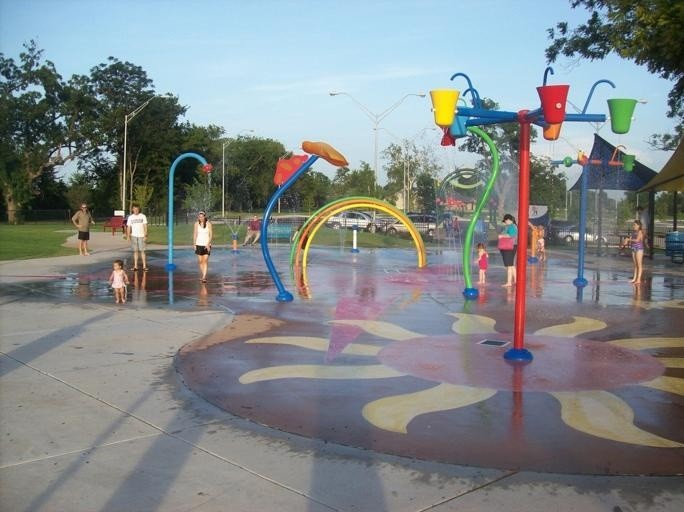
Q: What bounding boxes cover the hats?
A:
[502,214,511,221]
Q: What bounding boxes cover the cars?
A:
[554,226,607,244]
[381,213,439,237]
[326,212,385,233]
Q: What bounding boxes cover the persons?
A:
[452,218,460,235]
[130,271,148,307]
[192,211,212,283]
[631,283,642,312]
[199,281,208,308]
[241,215,261,246]
[504,286,516,305]
[474,242,489,284]
[497,214,517,287]
[125,204,149,272]
[622,219,643,286]
[536,223,546,262]
[71,204,95,256]
[535,261,546,299]
[636,206,647,238]
[108,260,130,303]
[291,218,308,250]
[476,284,487,311]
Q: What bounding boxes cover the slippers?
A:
[200,276,207,282]
[131,267,150,271]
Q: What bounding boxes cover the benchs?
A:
[664,231,684,263]
[246,222,293,244]
[103,216,126,236]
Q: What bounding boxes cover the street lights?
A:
[221,129,255,217]
[123,94,170,211]
[567,96,648,137]
[329,90,436,219]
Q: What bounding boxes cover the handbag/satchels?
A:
[498,238,514,250]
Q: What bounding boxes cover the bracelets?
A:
[144,235,147,237]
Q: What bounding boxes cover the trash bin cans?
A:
[666,231,684,256]
